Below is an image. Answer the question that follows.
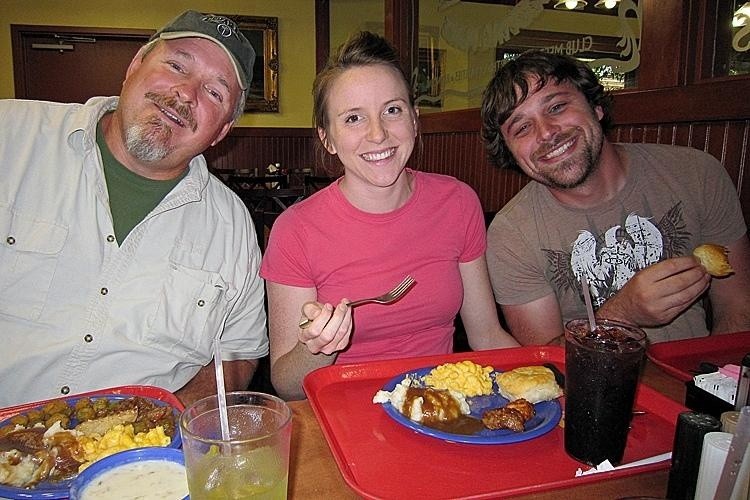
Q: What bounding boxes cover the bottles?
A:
[661,352,749,500]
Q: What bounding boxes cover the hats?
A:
[148,9,257,103]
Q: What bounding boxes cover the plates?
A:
[0,394,182,499]
[381,365,565,447]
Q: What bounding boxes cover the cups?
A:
[178,389,294,500]
[563,317,651,469]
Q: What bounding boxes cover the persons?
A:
[0,10,269,416]
[476,49,750,348]
[258,30,522,403]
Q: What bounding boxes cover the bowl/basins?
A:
[67,446,191,499]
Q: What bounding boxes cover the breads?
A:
[693,243,734,279]
[493,365,565,407]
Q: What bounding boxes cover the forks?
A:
[298,271,417,334]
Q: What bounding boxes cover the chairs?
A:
[208,167,339,252]
